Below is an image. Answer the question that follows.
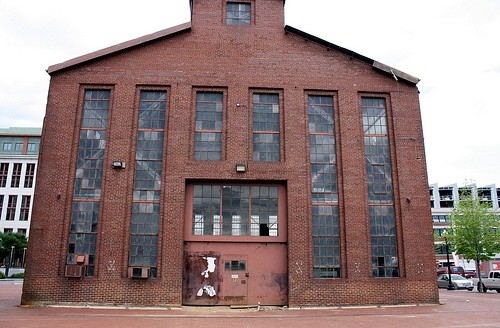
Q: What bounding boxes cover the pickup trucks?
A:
[473,270,500,293]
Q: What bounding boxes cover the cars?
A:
[437,273,474,291]
[436,265,477,279]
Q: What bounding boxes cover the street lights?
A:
[9,245,15,267]
[442,229,454,291]
[22,247,27,269]
